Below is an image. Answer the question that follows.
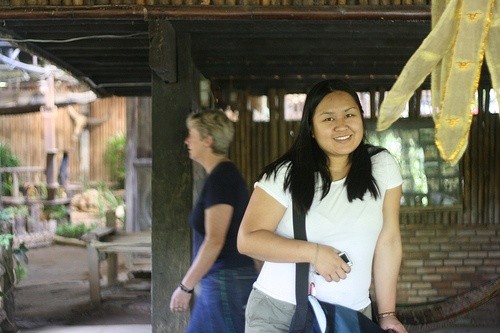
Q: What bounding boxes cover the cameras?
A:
[316,252,353,281]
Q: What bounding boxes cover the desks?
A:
[82,211,152,304]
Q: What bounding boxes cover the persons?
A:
[236,79,408,333]
[169,110,258,333]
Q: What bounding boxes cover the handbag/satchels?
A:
[290,297,387,333]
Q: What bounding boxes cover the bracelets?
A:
[378,312,397,318]
[178,282,194,294]
